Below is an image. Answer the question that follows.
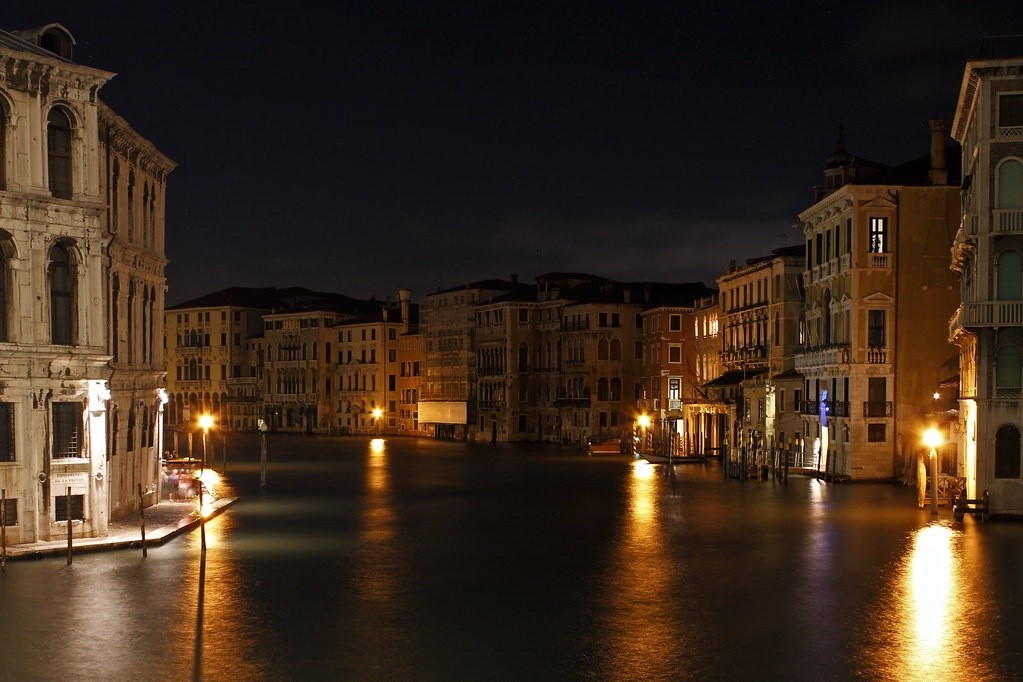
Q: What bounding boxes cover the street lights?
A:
[924,429,943,514]
[639,415,648,453]
